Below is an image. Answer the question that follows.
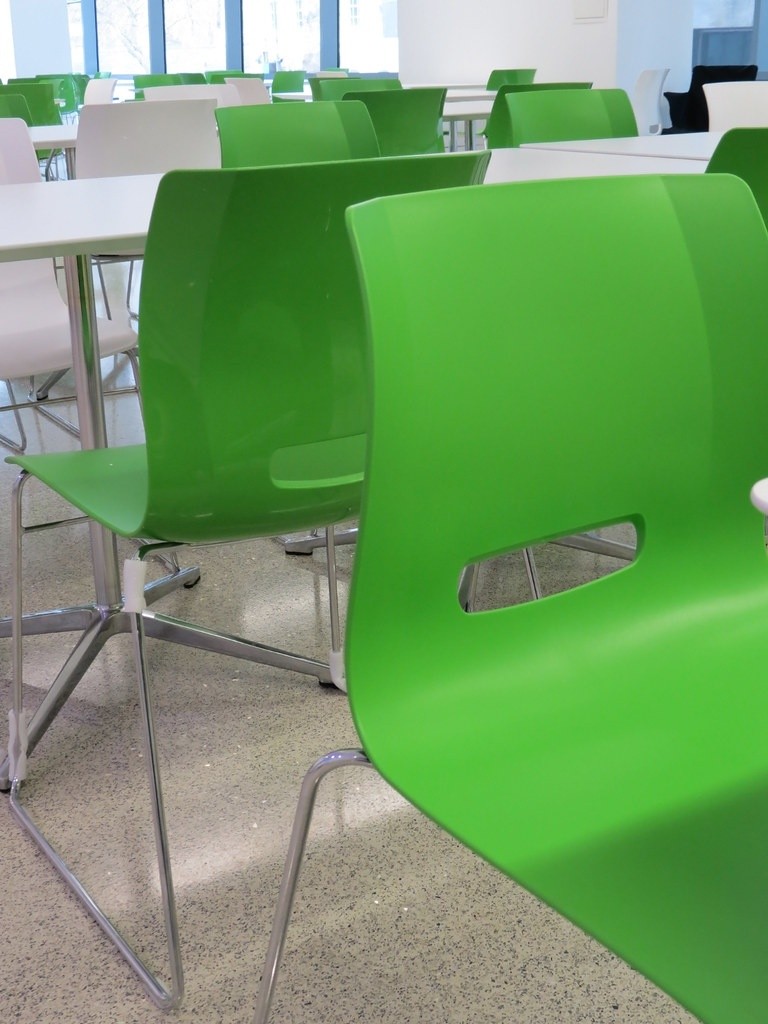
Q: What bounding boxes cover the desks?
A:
[25,124,80,179]
[274,90,314,101]
[520,133,728,162]
[3,156,336,790]
[438,100,499,152]
[443,87,498,151]
[484,148,712,188]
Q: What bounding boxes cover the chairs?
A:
[2,69,767,1021]
